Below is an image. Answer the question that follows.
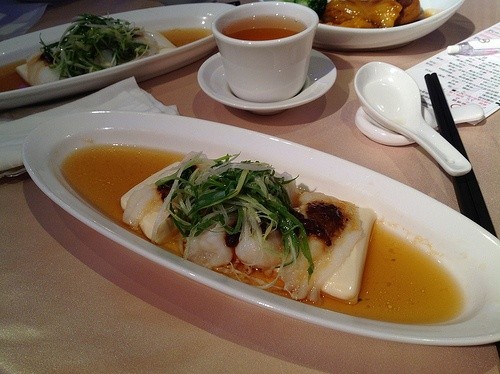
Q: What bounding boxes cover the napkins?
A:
[0,75,180,171]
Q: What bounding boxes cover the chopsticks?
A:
[424,72,498,239]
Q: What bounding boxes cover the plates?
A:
[20,110,500,348]
[0,2,237,109]
[196,49,338,114]
[259,0,467,50]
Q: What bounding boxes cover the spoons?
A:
[353,62,473,177]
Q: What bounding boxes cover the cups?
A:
[211,1,321,102]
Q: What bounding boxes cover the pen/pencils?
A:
[447,38,500,55]
